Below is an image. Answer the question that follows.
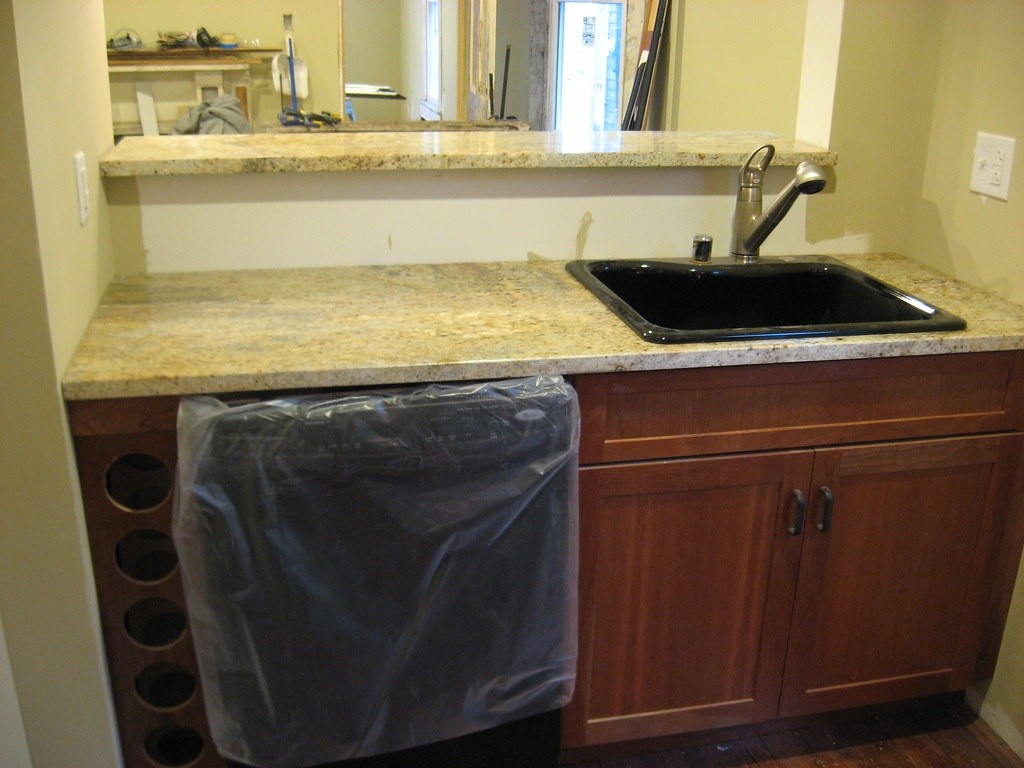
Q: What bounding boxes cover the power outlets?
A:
[969,131,1017,200]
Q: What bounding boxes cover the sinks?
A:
[564,254,968,345]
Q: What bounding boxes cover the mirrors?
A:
[102,0,809,147]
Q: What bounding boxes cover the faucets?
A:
[729,143,828,259]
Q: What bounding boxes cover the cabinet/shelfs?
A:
[560,348,1024,768]
